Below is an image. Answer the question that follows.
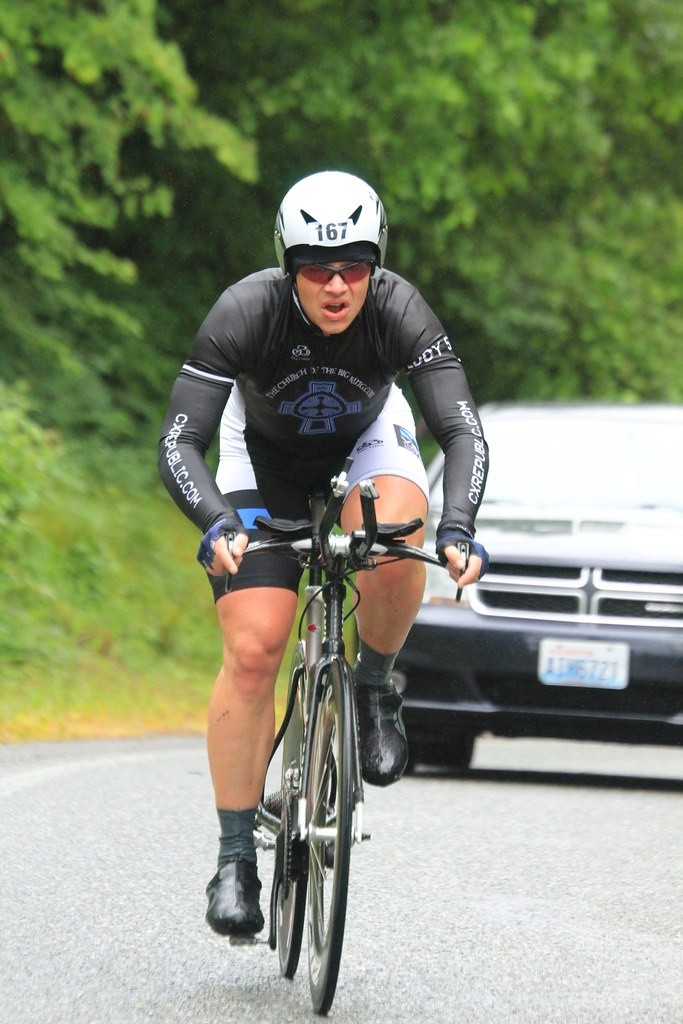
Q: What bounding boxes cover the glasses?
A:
[299,261,370,284]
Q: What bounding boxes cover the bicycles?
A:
[224,473,475,1005]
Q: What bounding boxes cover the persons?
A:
[156,172,491,934]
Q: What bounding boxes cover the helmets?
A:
[274,171,388,275]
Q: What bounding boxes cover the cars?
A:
[383,405,683,764]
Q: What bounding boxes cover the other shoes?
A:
[204,860,266,934]
[355,684,408,787]
